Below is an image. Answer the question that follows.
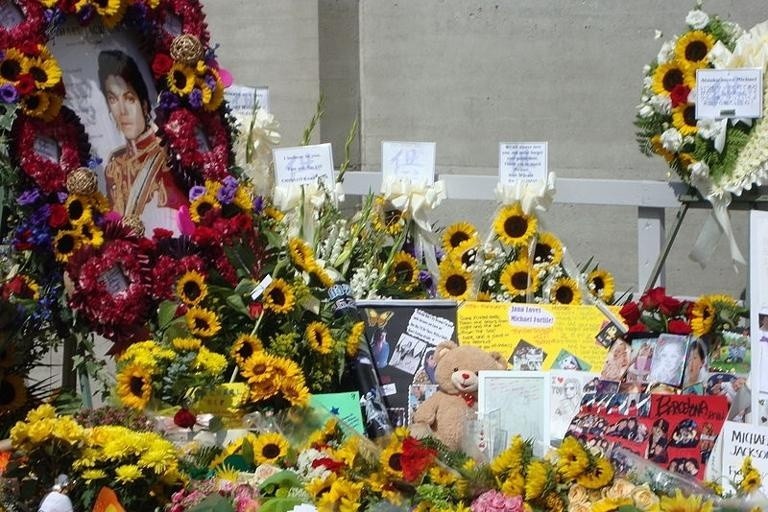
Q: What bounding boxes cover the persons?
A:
[709,378,747,418]
[570,394,717,477]
[709,328,750,364]
[760,312,768,331]
[603,342,628,380]
[98,50,192,240]
[601,327,621,345]
[632,343,654,376]
[371,329,390,368]
[688,341,705,386]
[651,336,685,382]
[414,349,437,385]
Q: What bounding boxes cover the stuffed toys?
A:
[408,341,509,451]
